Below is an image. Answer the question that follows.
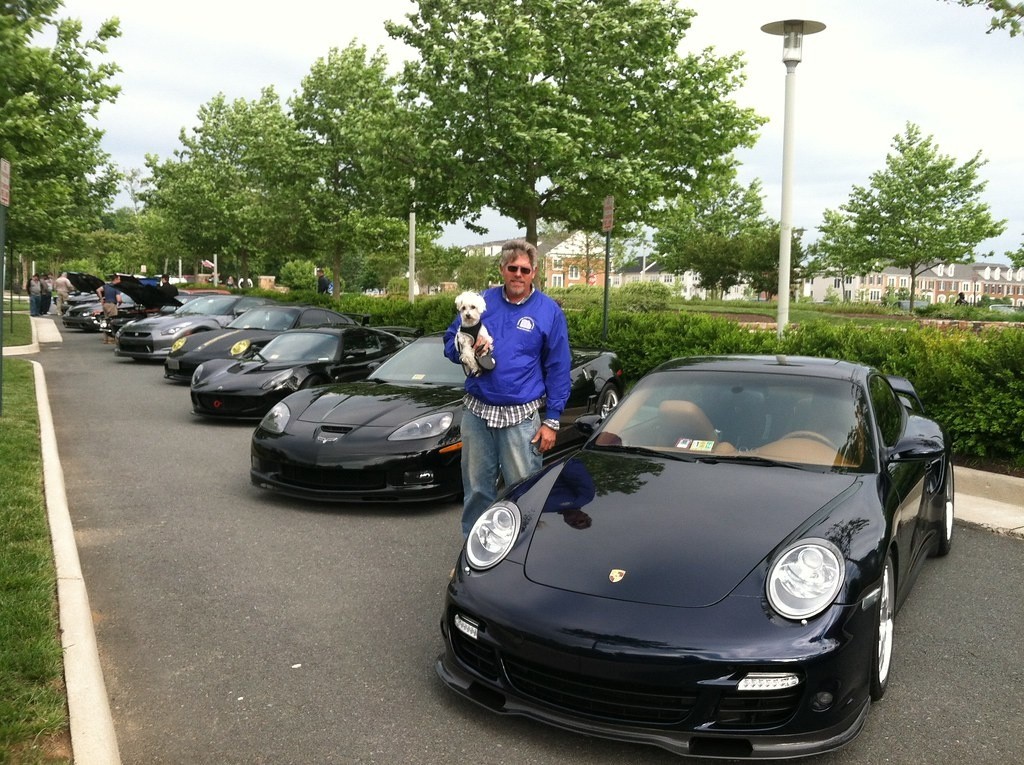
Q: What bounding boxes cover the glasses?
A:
[506,266,532,274]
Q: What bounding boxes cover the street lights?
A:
[759,18,825,336]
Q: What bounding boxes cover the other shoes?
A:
[104,338,116,344]
[448,568,456,579]
[31,312,47,317]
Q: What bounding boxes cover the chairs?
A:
[786,396,864,462]
[657,399,721,451]
[270,312,287,328]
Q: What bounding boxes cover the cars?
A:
[54,271,232,334]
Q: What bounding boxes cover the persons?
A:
[26,272,54,317]
[209,272,222,285]
[955,292,969,306]
[54,272,74,315]
[227,276,234,287]
[160,274,179,296]
[443,239,572,541]
[96,274,122,344]
[317,270,329,294]
[238,277,253,289]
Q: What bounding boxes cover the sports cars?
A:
[435,350,957,763]
[250,336,630,510]
[162,301,363,384]
[113,293,277,361]
[189,322,412,427]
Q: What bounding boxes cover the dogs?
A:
[455,290,495,377]
